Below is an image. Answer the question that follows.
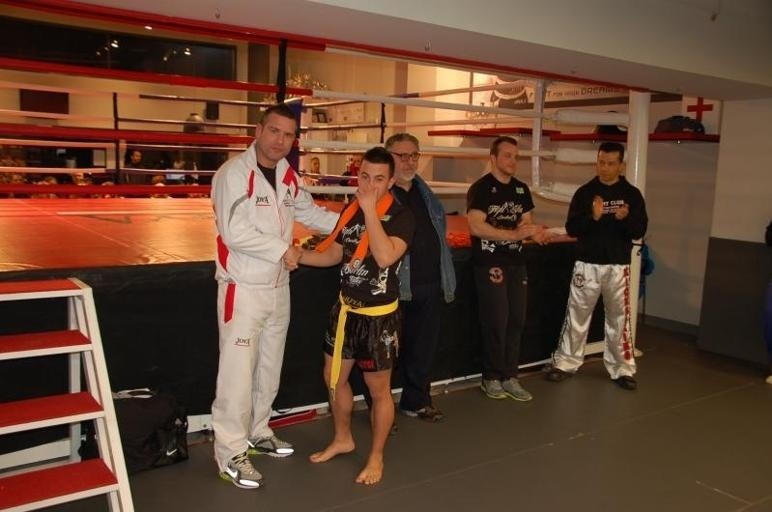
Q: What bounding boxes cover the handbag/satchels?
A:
[86,388,189,476]
[654,116,705,135]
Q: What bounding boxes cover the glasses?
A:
[392,151,420,160]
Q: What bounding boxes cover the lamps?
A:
[183,68,210,137]
[263,49,333,109]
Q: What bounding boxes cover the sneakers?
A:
[609,375,636,390]
[502,376,533,401]
[547,368,572,381]
[481,378,507,399]
[400,401,444,422]
[247,434,296,458]
[218,452,264,490]
[367,409,399,435]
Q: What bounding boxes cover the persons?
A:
[763,218,771,386]
[465,138,554,403]
[361,127,458,433]
[209,103,341,491]
[545,141,650,391]
[288,147,417,486]
[0,145,364,200]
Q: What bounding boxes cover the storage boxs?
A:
[678,95,726,137]
